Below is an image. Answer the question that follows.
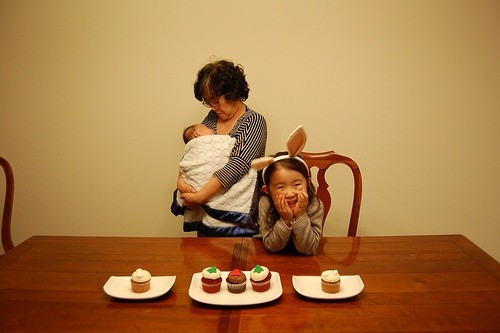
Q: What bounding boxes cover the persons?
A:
[247,125,324,256]
[171,59,267,236]
[183,125,216,143]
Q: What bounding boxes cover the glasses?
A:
[202,95,223,108]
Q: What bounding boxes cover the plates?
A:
[188,271,283,306]
[292,275,364,300]
[103,275,176,299]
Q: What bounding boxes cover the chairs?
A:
[0,156,14,255]
[299,151,363,237]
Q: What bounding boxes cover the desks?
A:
[0,235,500,333]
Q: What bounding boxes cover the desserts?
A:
[321,269,341,293]
[250,265,272,292]
[131,268,151,293]
[226,269,247,293]
[201,265,222,293]
